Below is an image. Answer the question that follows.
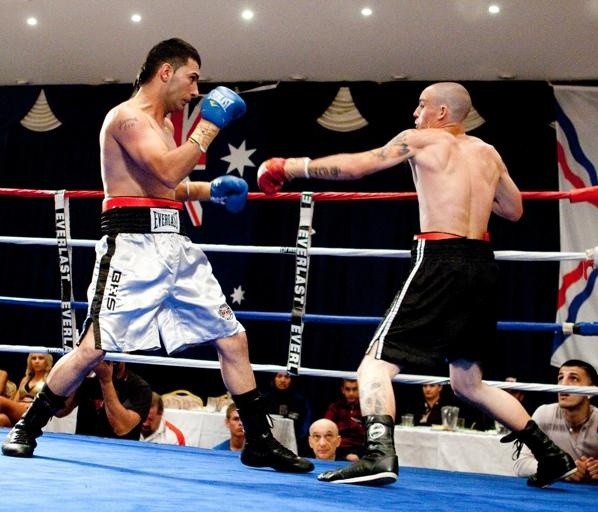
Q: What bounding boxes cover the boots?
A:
[500,420,577,488]
[2,382,70,457]
[317,415,399,486]
[231,389,315,473]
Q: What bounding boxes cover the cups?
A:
[401,406,508,435]
[208,397,220,411]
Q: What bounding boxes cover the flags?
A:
[164,94,207,227]
[550,82,597,374]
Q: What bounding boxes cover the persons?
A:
[257,83,578,488]
[2,38,314,474]
[0,350,598,482]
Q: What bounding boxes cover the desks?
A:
[41,405,299,456]
[394,424,523,476]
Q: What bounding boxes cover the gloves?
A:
[200,86,246,128]
[209,175,248,213]
[257,157,288,195]
